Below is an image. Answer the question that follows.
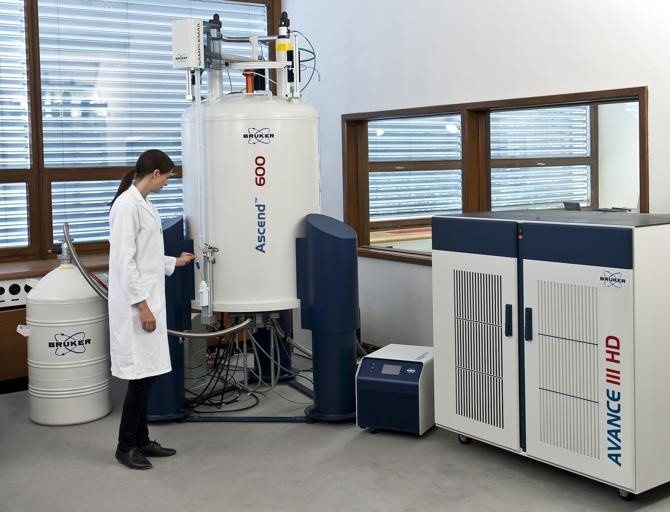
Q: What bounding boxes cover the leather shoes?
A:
[116,445,152,470]
[141,441,176,457]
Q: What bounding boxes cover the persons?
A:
[108,149,196,468]
